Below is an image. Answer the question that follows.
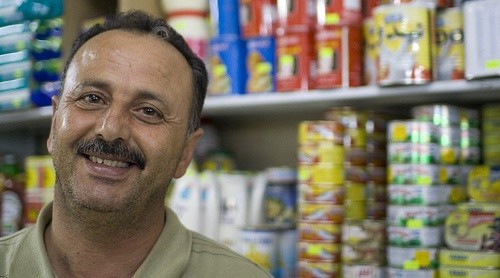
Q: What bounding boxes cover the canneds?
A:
[296,105,500,277]
[159,0,500,98]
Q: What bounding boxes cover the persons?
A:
[0,9,275,278]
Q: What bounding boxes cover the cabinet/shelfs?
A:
[0,0,500,278]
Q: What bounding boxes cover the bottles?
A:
[0,154,24,237]
